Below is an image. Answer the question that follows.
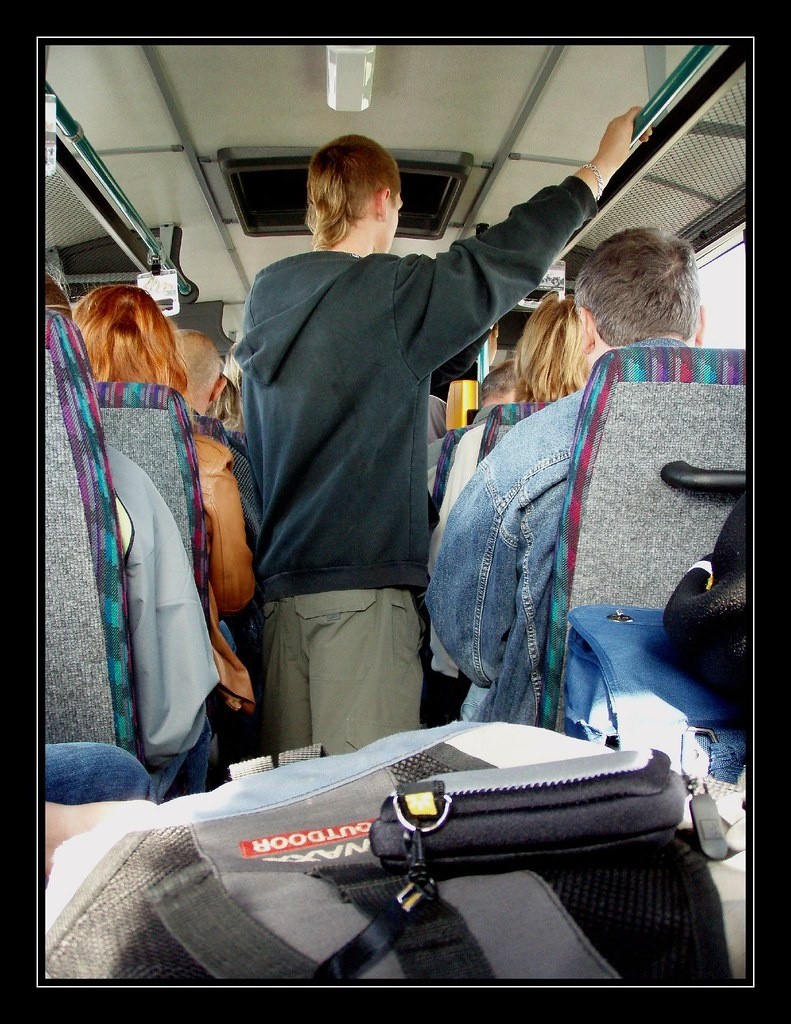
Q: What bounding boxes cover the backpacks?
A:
[46,718,734,979]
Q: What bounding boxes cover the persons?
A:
[173,329,260,536]
[426,358,517,499]
[427,290,589,679]
[44,274,221,805]
[45,741,158,881]
[232,108,653,757]
[424,227,706,728]
[72,284,256,714]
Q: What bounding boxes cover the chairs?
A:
[434,347,744,725]
[45,310,211,780]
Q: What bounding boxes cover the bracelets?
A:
[579,164,604,201]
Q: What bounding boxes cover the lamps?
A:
[327,44,376,113]
